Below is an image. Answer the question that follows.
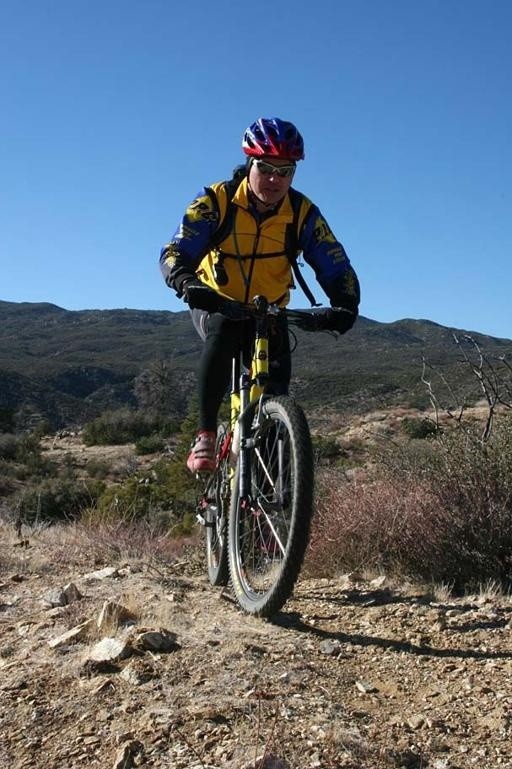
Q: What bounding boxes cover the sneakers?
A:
[249,512,281,555]
[187,435,217,473]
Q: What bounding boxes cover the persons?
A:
[158,118,360,475]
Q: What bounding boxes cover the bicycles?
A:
[195,293,340,620]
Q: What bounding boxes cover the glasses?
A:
[251,157,297,178]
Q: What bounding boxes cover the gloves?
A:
[300,309,350,332]
[181,278,221,315]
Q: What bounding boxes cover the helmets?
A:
[241,117,305,160]
